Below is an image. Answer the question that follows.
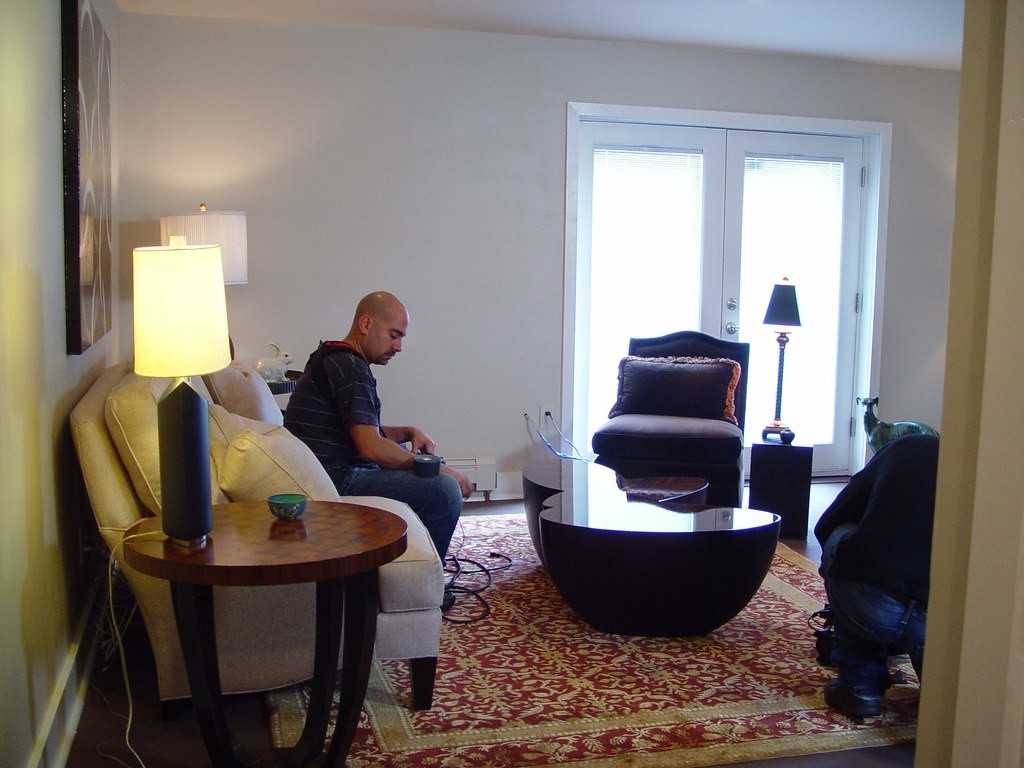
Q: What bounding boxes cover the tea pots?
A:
[780,427,795,444]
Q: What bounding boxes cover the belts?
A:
[880,585,927,610]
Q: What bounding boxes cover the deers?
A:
[856,396,939,455]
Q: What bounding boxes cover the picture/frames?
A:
[61,0,112,356]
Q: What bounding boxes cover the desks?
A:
[123,500,408,768]
[522,456,781,637]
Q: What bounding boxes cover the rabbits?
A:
[253,341,295,383]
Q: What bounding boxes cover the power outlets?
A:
[540,405,556,430]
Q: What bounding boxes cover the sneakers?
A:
[824,678,881,718]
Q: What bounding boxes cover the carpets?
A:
[265,512,917,767]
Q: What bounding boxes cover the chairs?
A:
[591,331,750,507]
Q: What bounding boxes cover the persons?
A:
[283,291,475,612]
[814,434,940,718]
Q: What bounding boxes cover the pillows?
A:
[209,405,342,503]
[608,355,741,426]
[202,362,284,426]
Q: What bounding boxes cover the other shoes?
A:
[440,591,456,610]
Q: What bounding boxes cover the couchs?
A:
[69,360,445,722]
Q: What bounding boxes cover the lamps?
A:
[132,236,232,546]
[159,203,248,285]
[762,277,802,440]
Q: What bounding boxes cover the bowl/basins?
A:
[267,494,307,521]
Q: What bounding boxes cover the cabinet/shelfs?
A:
[749,441,814,540]
